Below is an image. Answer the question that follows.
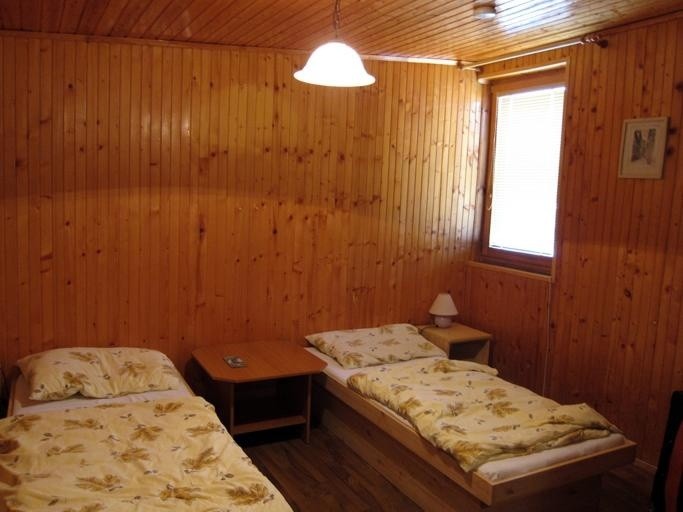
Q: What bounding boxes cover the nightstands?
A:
[191,339,328,445]
[414,321,491,366]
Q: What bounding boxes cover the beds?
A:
[0,346,295,512]
[302,323,638,511]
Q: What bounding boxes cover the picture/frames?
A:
[617,117,668,179]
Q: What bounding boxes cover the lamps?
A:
[293,1,376,88]
[430,293,458,328]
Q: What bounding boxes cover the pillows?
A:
[303,323,447,369]
[12,346,179,402]
